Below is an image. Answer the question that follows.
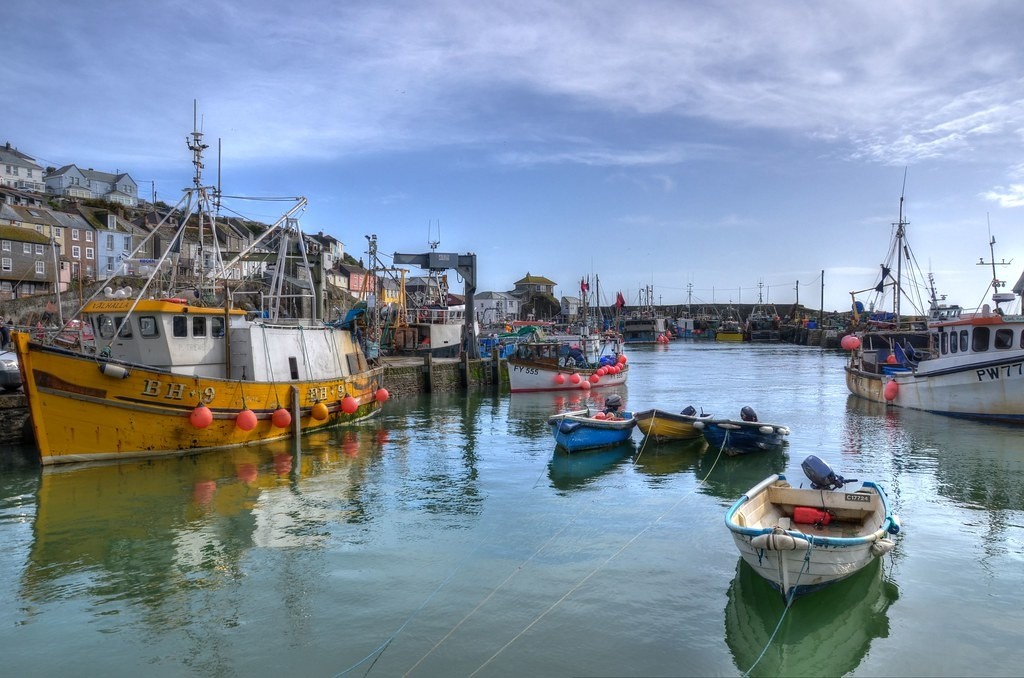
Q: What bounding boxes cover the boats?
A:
[841,165,1024,425]
[724,454,892,608]
[633,405,715,444]
[548,394,637,454]
[693,406,791,457]
[11,98,385,465]
[624,277,781,344]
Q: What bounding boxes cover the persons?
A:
[7,318,13,325]
[0,315,10,351]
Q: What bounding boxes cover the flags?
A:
[581,274,590,291]
[617,292,625,308]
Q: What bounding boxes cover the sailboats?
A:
[506,272,629,393]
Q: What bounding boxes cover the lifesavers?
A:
[420,306,430,318]
[154,297,189,304]
[368,327,382,341]
